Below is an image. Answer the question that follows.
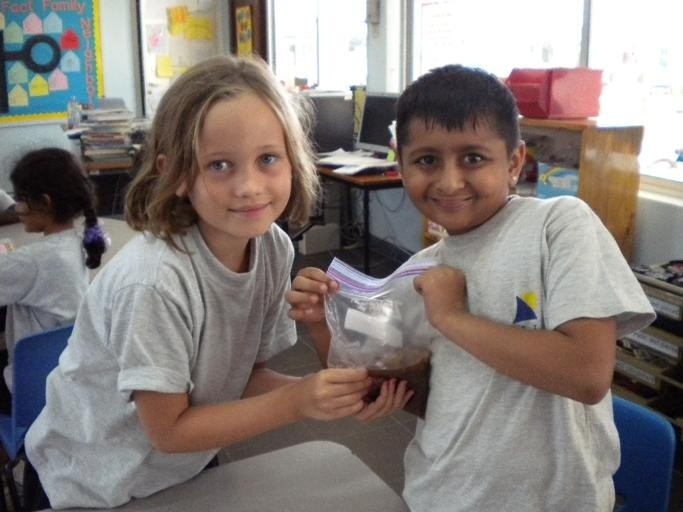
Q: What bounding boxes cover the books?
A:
[65,92,138,159]
[608,255,682,424]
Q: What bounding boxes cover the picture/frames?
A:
[230,1,260,56]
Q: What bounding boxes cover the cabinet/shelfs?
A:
[614,258,682,413]
[422,118,644,270]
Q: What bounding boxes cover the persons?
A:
[0,149,108,418]
[21,58,417,511]
[285,63,655,510]
[0,187,19,225]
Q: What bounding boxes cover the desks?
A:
[312,160,415,276]
[81,158,134,176]
[0,216,141,284]
[38,438,409,511]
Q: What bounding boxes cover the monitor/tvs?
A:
[356,94,400,154]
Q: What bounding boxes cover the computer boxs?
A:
[309,96,354,152]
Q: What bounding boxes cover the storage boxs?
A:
[506,66,602,121]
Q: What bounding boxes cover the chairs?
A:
[0,323,75,511]
[610,396,676,510]
